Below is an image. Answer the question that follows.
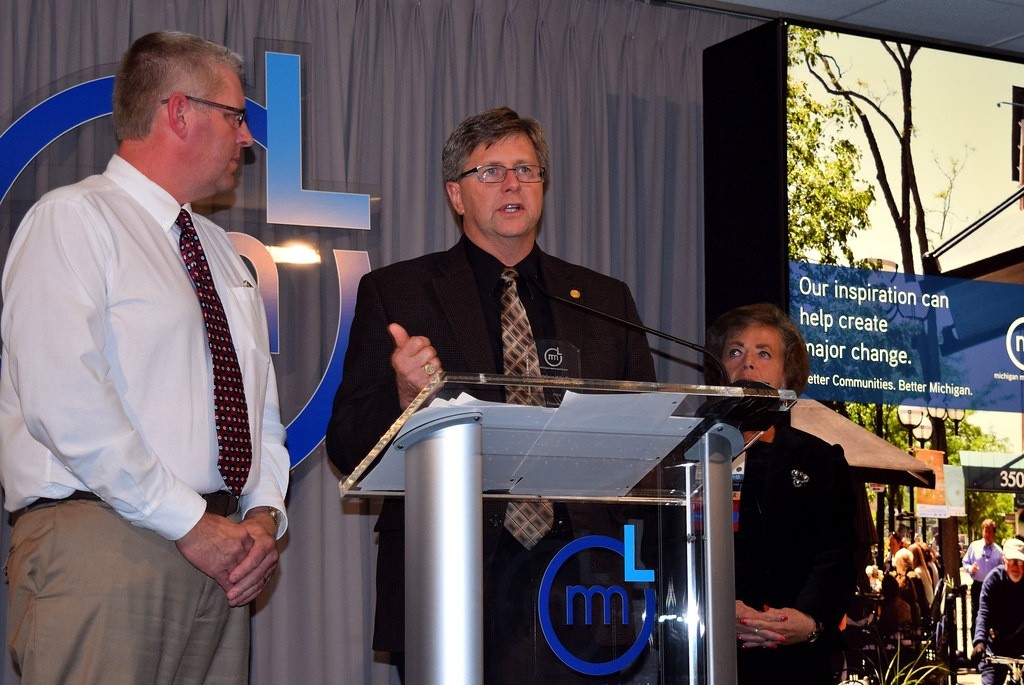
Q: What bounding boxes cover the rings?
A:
[424,362,436,375]
[752,628,758,635]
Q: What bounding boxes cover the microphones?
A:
[519,259,781,433]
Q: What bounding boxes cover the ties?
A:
[499,266,554,553]
[175,209,253,500]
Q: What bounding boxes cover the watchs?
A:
[806,622,824,643]
[248,507,281,527]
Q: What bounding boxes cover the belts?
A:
[9,493,238,517]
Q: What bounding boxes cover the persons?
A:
[971,538,1024,685]
[962,518,1005,640]
[641,304,866,685]
[327,105,656,685]
[0,30,290,685]
[879,531,940,635]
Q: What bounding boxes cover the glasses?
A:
[161,96,247,127]
[456,165,545,183]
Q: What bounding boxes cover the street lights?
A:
[912,417,933,544]
[898,397,923,545]
[948,395,966,436]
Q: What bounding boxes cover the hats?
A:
[1003,539,1024,562]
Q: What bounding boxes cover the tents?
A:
[791,400,936,570]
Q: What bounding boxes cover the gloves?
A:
[971,642,985,665]
